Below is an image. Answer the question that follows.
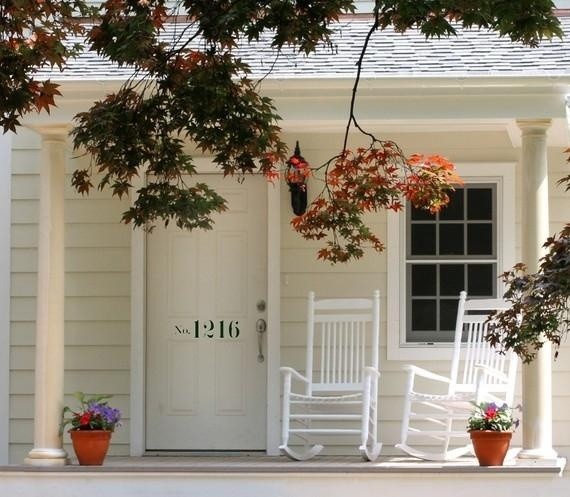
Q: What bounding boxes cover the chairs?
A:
[393,291,523,461]
[279,290,385,462]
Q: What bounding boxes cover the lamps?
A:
[285,138,309,217]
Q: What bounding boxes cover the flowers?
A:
[465,402,522,430]
[58,391,123,434]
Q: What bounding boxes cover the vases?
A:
[471,431,511,466]
[69,427,113,468]
